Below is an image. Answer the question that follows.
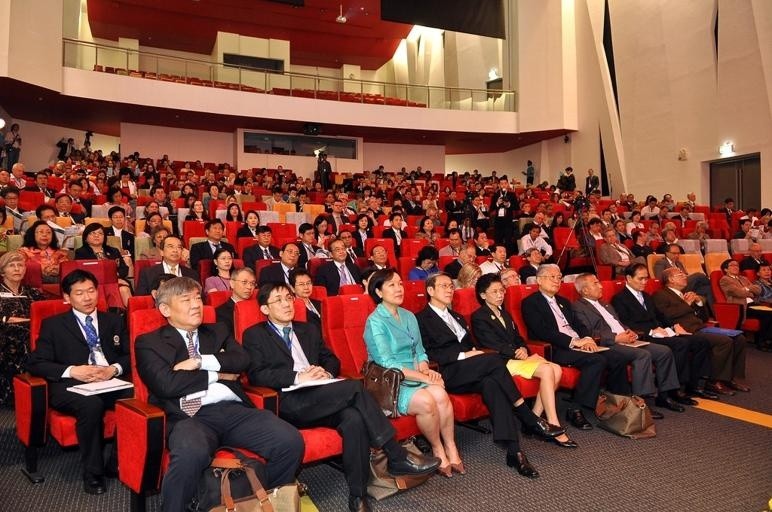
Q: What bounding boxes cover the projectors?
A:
[336,16,346,22]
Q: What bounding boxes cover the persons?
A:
[0,251,46,408]
[571,273,683,419]
[612,263,720,407]
[521,264,627,431]
[134,277,304,512]
[1,122,772,353]
[239,280,441,512]
[652,267,750,397]
[414,273,565,479]
[362,268,466,478]
[473,274,578,449]
[32,269,134,495]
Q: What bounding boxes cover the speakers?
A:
[304,125,320,134]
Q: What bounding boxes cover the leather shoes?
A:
[347,411,592,512]
[645,342,771,419]
[83,466,117,494]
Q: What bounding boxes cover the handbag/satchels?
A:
[182,460,301,512]
[360,360,405,419]
[595,389,657,440]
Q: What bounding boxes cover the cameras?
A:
[500,189,509,202]
[573,195,591,210]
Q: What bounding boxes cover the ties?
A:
[349,249,357,263]
[179,331,201,418]
[340,264,347,287]
[215,244,221,250]
[171,266,176,275]
[264,249,271,260]
[86,317,97,366]
[283,327,291,352]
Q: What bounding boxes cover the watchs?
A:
[111,365,118,377]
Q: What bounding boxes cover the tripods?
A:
[557,208,598,276]
